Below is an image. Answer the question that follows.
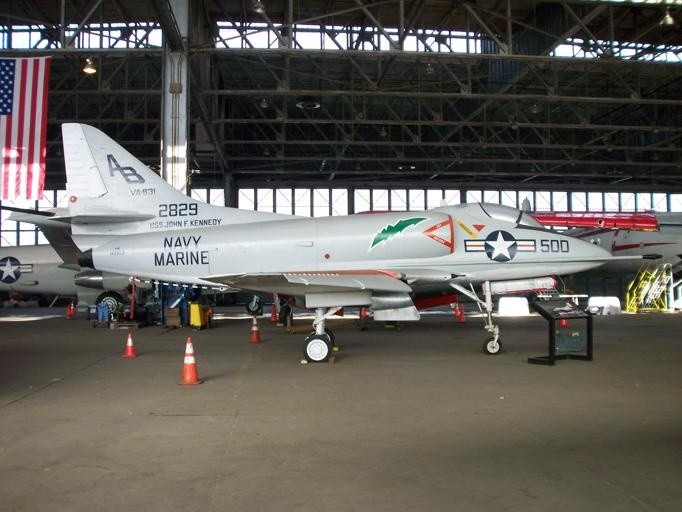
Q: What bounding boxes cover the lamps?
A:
[660,4,674,26]
[259,99,269,109]
[82,54,98,75]
[424,63,434,76]
[251,0,264,13]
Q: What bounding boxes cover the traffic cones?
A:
[178,336,203,385]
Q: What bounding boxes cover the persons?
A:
[279,296,296,330]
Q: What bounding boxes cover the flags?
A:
[0,54,52,203]
